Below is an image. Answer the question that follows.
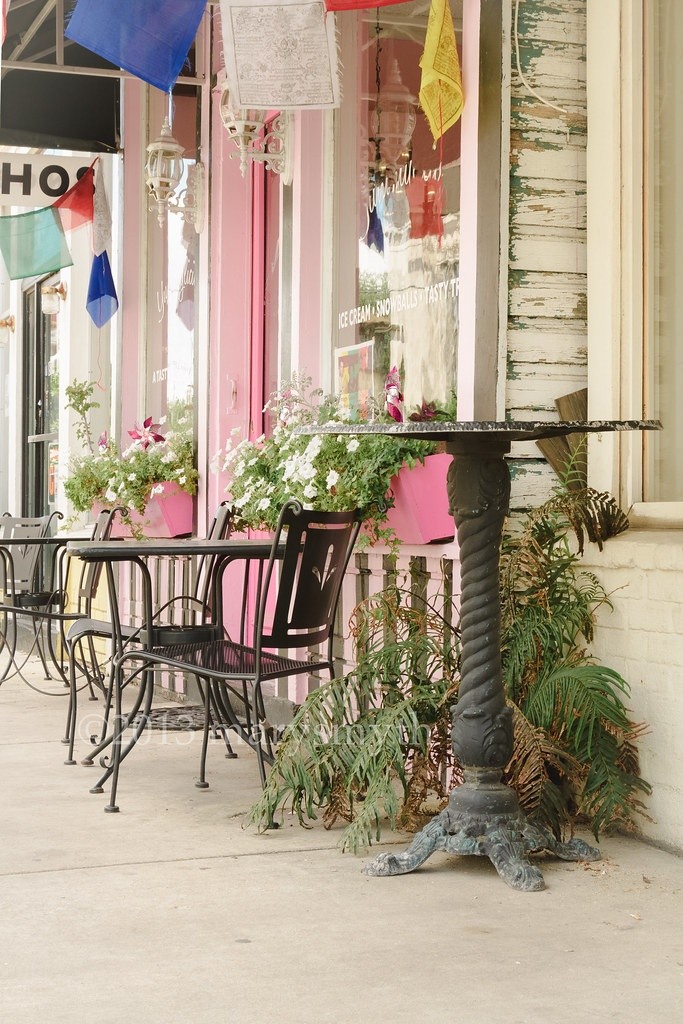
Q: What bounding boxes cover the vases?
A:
[91,481,193,538]
[302,453,456,545]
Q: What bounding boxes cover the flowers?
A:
[209,366,458,554]
[55,377,200,543]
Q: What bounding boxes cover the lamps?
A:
[145,116,204,234]
[218,78,294,186]
[40,281,68,314]
[0,314,15,349]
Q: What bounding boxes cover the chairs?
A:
[0,511,64,623]
[61,500,242,765]
[104,499,367,814]
[0,507,128,708]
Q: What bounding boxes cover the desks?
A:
[65,539,305,793]
[0,536,126,688]
[292,419,664,894]
